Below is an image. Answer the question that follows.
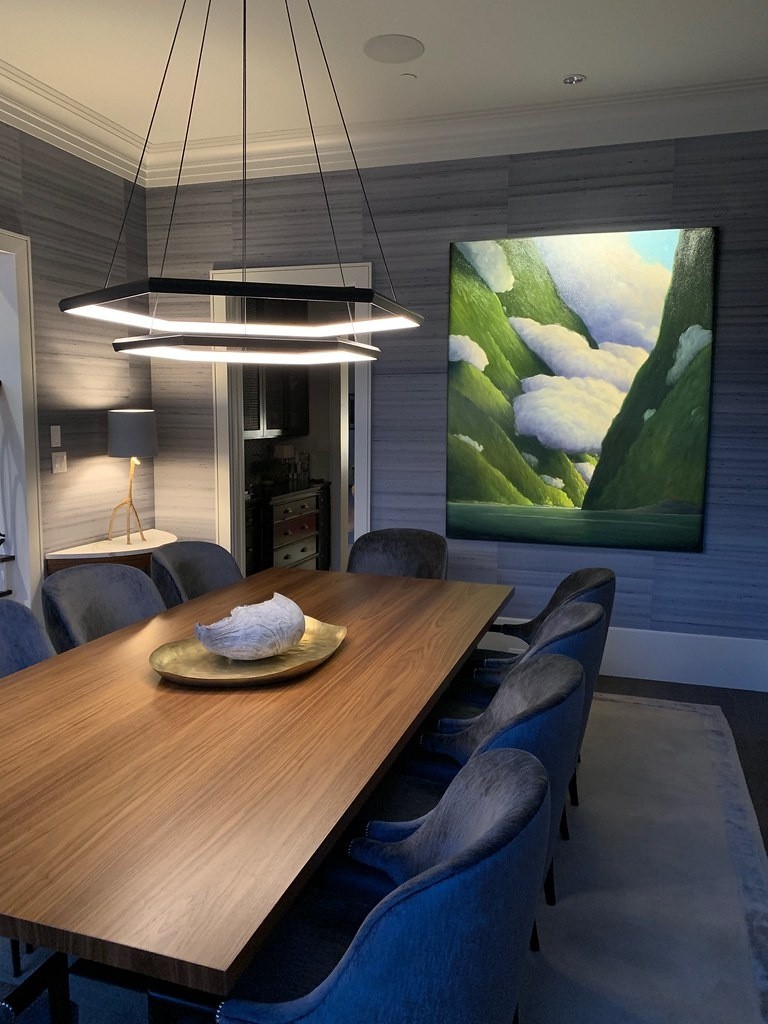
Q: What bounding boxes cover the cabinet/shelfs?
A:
[262,364,310,439]
[261,481,331,571]
[242,363,264,440]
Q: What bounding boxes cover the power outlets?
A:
[51,425,61,447]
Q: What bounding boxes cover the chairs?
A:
[40,563,166,655]
[334,652,585,905]
[420,567,616,763]
[0,951,79,1024]
[346,527,448,580]
[405,600,607,806]
[150,542,244,609]
[0,598,53,678]
[214,748,552,1024]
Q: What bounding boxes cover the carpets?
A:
[0,692,768,1024]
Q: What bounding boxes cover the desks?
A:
[0,567,515,997]
[46,528,178,575]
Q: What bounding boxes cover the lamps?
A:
[59,0,425,368]
[273,444,295,480]
[106,408,158,545]
